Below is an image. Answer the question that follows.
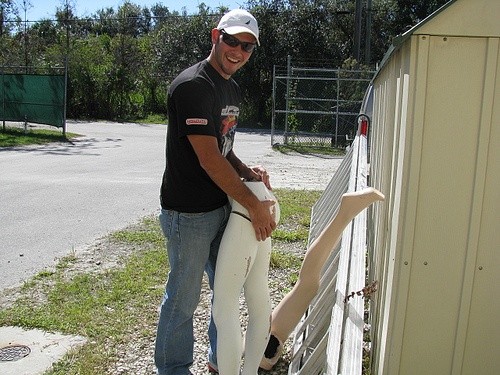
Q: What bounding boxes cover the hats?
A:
[217,8,261,46]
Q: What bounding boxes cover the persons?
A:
[257,183,396,372]
[150,6,280,374]
[209,177,276,374]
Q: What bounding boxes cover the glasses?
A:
[221,31,256,52]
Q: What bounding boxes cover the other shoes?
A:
[208,362,241,375]
